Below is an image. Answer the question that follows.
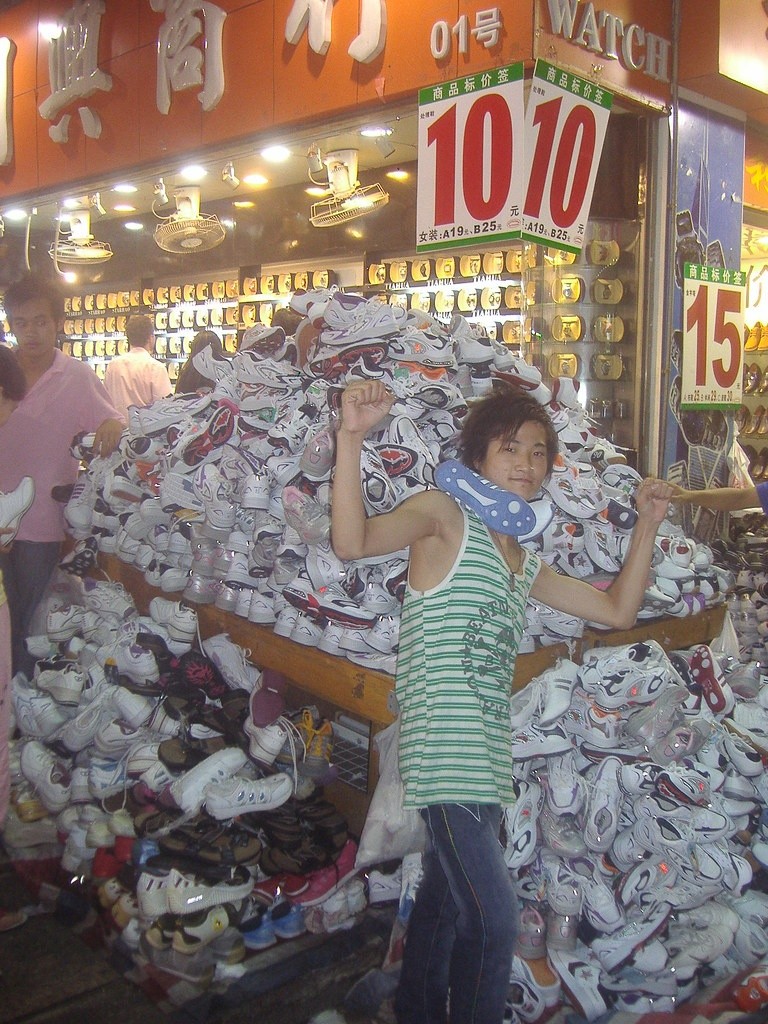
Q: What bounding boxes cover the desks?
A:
[117,563,727,843]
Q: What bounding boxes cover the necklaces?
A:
[494,532,521,591]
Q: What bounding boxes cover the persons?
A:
[0,272,301,933]
[328,377,677,1024]
[640,477,768,512]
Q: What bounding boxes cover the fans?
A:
[153,186,224,255]
[309,149,390,227]
[48,209,113,264]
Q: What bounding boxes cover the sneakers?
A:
[0,288,768,1024]
